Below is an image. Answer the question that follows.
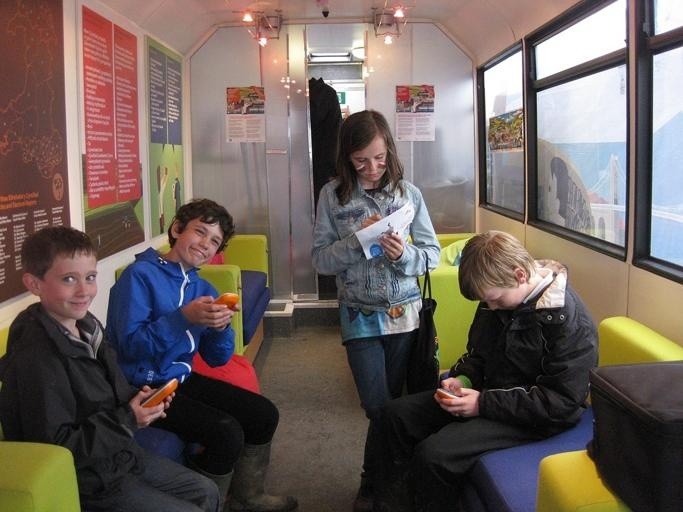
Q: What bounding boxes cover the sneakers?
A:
[353,493,373,511]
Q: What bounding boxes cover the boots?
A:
[182,454,233,511]
[232,441,298,511]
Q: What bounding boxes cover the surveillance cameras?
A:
[322,10,329,18]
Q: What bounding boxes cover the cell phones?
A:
[436,388,460,400]
[212,292,239,309]
[139,378,178,407]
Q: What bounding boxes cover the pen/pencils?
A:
[365,206,371,218]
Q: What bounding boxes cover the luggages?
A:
[586,362,682,512]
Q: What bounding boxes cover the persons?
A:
[103,198,296,512]
[1,225,220,512]
[313,110,439,423]
[353,229,597,512]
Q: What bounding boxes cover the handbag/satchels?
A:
[406,298,439,394]
